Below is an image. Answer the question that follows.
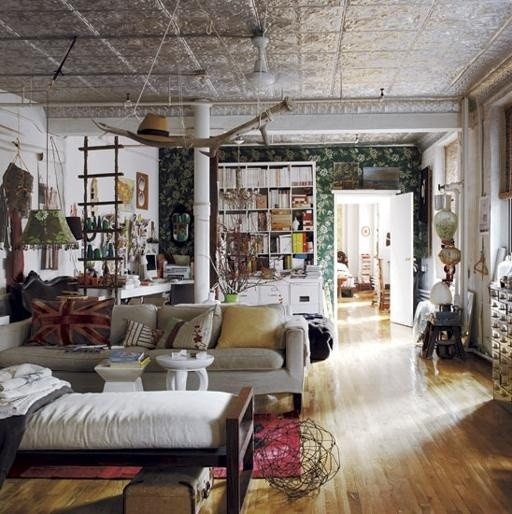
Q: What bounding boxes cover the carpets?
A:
[5,414,302,480]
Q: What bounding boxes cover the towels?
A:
[0,362,72,419]
[278,314,312,370]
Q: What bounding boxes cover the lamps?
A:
[12,90,81,251]
[218,138,264,214]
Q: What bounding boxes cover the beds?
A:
[337,251,353,300]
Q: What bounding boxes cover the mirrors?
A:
[169,202,194,246]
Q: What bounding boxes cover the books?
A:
[216,166,321,280]
[108,350,145,363]
[109,355,152,368]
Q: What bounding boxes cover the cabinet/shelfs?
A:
[216,160,318,267]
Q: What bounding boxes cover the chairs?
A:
[163,283,194,306]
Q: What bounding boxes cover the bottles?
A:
[87,211,114,259]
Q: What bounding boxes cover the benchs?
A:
[15,383,256,514]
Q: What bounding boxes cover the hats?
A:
[125,111,176,146]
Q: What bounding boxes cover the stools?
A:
[421,318,471,362]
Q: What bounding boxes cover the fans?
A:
[196,35,344,92]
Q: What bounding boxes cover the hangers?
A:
[474,251,488,275]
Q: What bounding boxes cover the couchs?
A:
[0,314,306,421]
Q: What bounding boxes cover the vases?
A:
[220,293,239,305]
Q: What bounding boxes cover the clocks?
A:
[361,226,370,237]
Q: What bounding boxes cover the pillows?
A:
[215,304,283,351]
[157,304,223,349]
[22,297,115,350]
